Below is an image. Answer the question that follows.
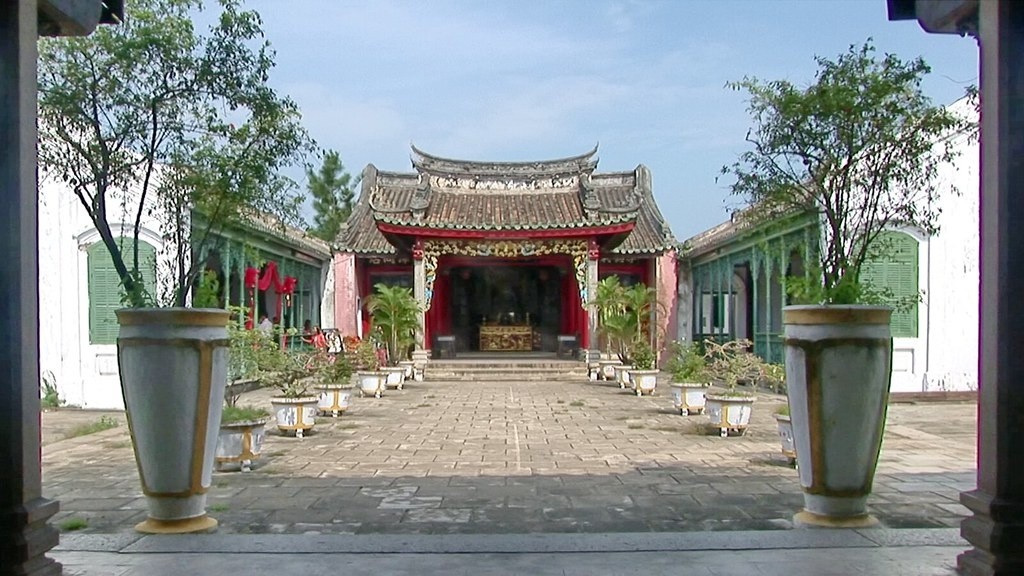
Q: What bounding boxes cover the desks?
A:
[479,326,532,352]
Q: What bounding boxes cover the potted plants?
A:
[589,35,981,530]
[32,0,425,534]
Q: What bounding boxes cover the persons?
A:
[300,325,330,352]
[258,312,311,334]
